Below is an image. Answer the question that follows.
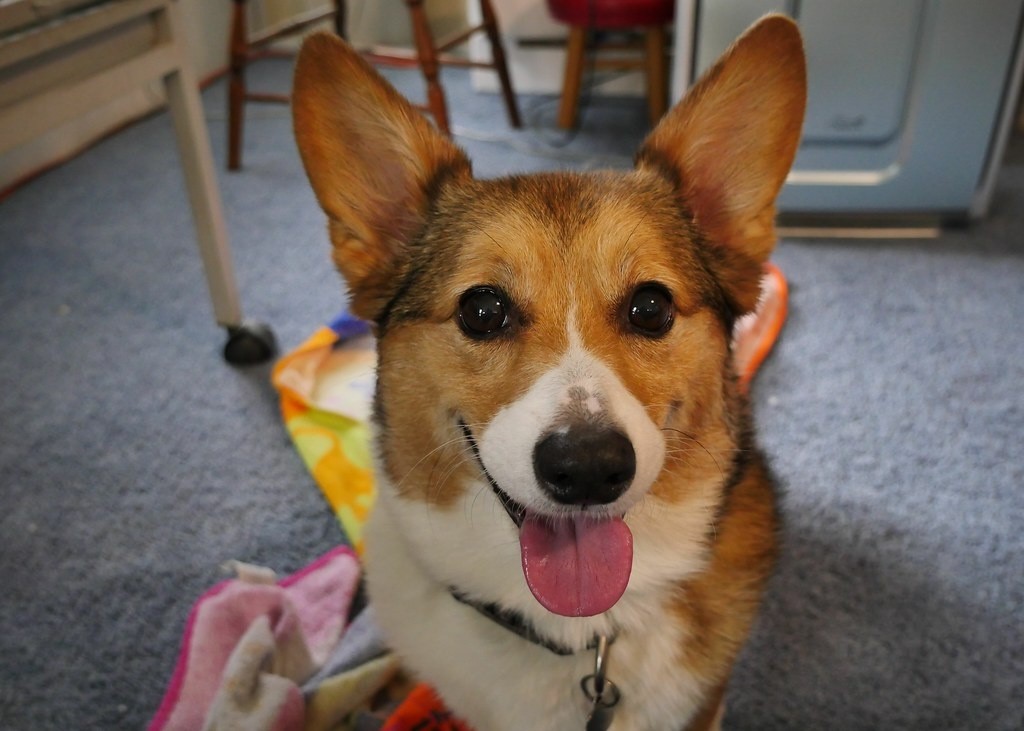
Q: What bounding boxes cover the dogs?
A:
[290,9,816,731]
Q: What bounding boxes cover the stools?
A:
[547,0,673,131]
